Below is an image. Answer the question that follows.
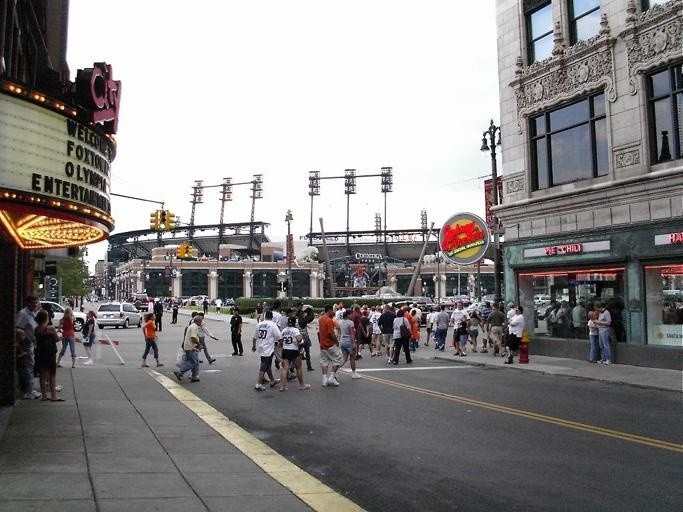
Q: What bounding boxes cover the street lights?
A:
[308,171,320,246]
[140,256,149,293]
[422,231,450,309]
[216,176,233,260]
[284,209,293,308]
[247,173,264,259]
[381,167,392,256]
[344,169,355,256]
[419,208,428,242]
[373,211,382,243]
[481,118,503,311]
[187,180,203,245]
[164,248,177,297]
[467,275,475,299]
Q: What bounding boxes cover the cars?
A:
[348,293,550,327]
[646,292,665,305]
[94,302,142,329]
[505,283,530,309]
[74,295,324,307]
[32,301,87,332]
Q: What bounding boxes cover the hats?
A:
[232,307,238,310]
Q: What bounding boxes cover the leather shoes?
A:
[239,352,243,356]
[191,377,200,383]
[176,371,183,380]
[232,350,238,355]
[407,359,413,363]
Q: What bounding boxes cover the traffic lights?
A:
[166,211,175,231]
[176,245,181,258]
[188,245,191,258]
[182,244,188,258]
[274,283,281,292]
[159,211,166,230]
[149,211,157,230]
[282,282,287,292]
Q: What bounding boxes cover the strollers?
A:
[275,343,305,381]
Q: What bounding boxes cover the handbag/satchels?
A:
[400,318,411,338]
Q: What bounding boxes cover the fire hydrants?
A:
[80,304,83,313]
[517,331,530,363]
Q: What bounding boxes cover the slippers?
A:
[298,383,311,390]
[41,396,51,401]
[50,396,65,402]
[279,386,289,392]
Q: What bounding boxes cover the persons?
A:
[15,293,223,402]
[316,300,422,388]
[422,295,525,365]
[252,300,314,390]
[621,309,626,329]
[230,307,244,356]
[533,296,612,366]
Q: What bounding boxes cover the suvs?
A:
[131,293,148,312]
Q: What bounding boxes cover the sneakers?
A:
[321,376,327,388]
[269,378,280,387]
[328,376,339,386]
[603,360,612,365]
[351,372,361,379]
[23,389,42,400]
[55,385,64,391]
[424,342,446,352]
[83,359,93,365]
[356,350,399,365]
[469,340,513,364]
[597,358,604,364]
[255,383,267,391]
[450,344,467,356]
